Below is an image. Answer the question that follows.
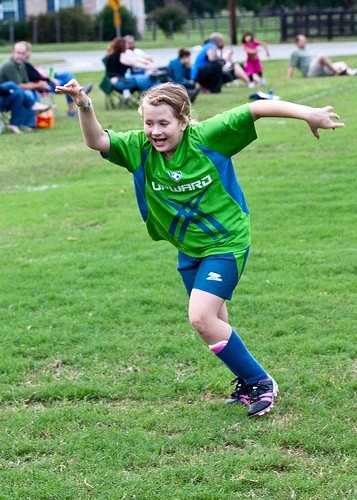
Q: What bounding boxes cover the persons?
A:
[55,79,344,416]
[0,32,269,135]
[286,34,357,77]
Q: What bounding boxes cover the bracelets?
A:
[79,98,91,112]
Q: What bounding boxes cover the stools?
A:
[0,111,9,136]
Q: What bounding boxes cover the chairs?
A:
[98,56,140,110]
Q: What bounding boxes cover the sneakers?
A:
[225,377,252,408]
[246,375,278,418]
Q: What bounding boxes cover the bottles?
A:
[48,67,55,78]
[0,122,4,133]
[5,120,9,135]
[124,68,131,81]
[267,89,274,100]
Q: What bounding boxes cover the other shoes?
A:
[337,66,357,76]
[82,83,93,94]
[32,104,52,114]
[66,108,78,116]
[6,126,24,135]
